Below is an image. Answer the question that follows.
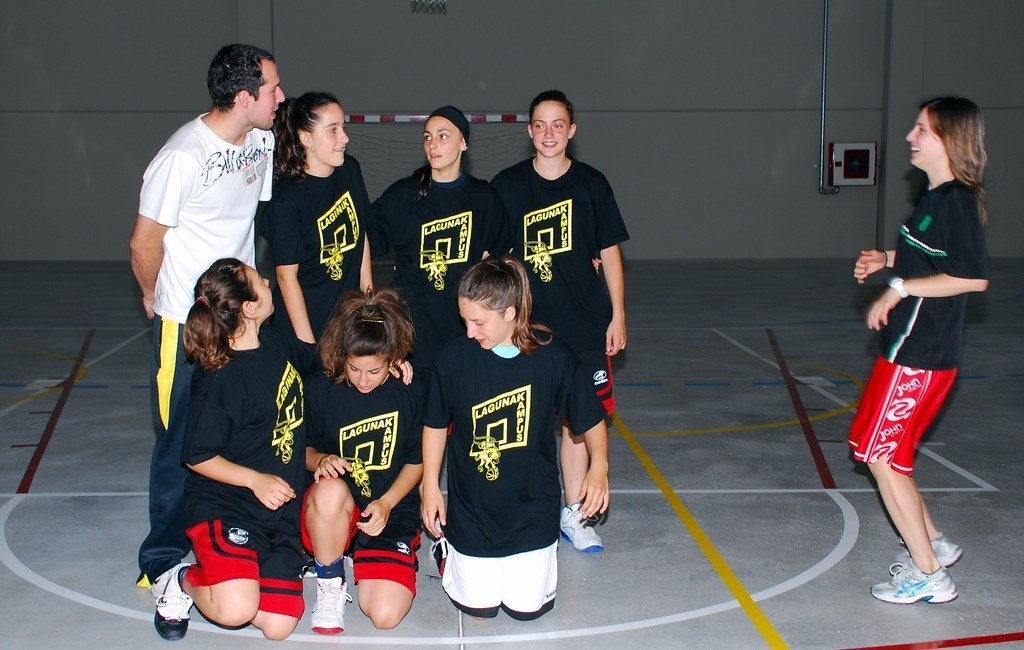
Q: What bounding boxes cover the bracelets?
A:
[880,250,887,269]
[317,456,328,467]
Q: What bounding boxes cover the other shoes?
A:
[301,556,317,577]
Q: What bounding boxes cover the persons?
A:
[372,106,507,580]
[299,287,423,633]
[129,44,286,590]
[489,91,628,552]
[849,96,990,603]
[421,257,609,620]
[154,257,306,639]
[255,91,378,579]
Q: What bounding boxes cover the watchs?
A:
[889,277,909,298]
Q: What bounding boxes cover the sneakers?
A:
[898,532,963,567]
[154,562,193,640]
[872,558,959,603]
[428,518,447,561]
[560,503,603,553]
[151,567,171,597]
[311,576,352,635]
